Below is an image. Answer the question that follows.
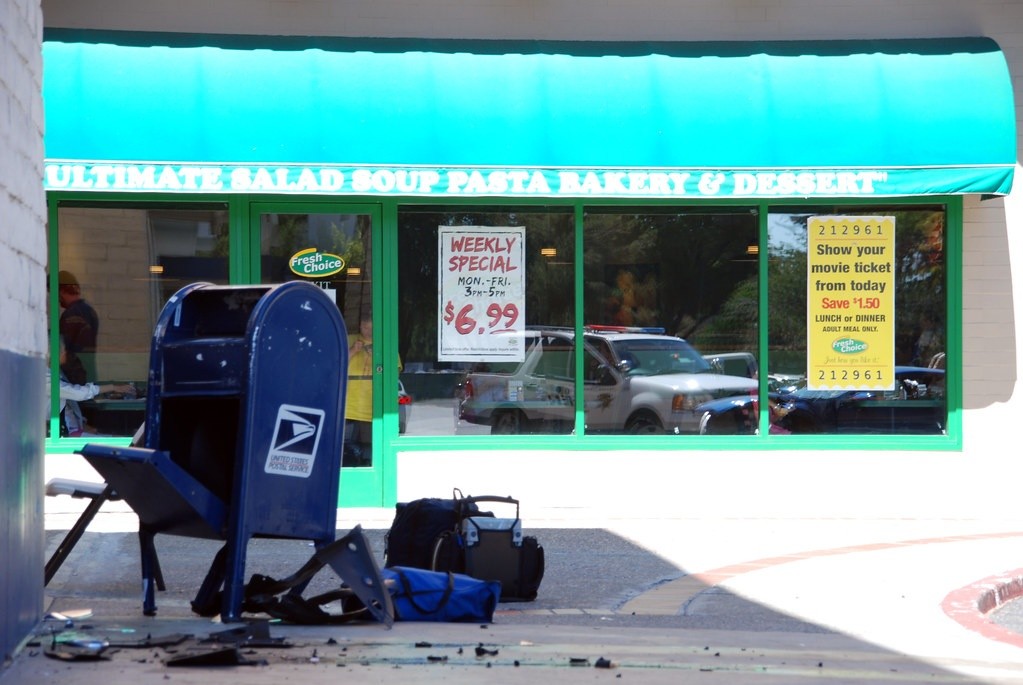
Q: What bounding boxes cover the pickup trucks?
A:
[455,324,763,434]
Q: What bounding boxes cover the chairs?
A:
[43,422,168,592]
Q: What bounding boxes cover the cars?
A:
[685,365,948,436]
[396,377,411,436]
[699,353,758,381]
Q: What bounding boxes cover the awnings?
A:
[45,35,1016,201]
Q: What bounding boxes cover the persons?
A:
[911,310,942,367]
[526,223,664,327]
[342,300,402,468]
[46,270,138,438]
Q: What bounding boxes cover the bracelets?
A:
[621,288,633,296]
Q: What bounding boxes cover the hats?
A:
[58,271,80,285]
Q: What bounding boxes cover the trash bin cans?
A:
[71,279,350,624]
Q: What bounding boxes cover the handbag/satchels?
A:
[380,564,503,623]
[384,489,544,602]
